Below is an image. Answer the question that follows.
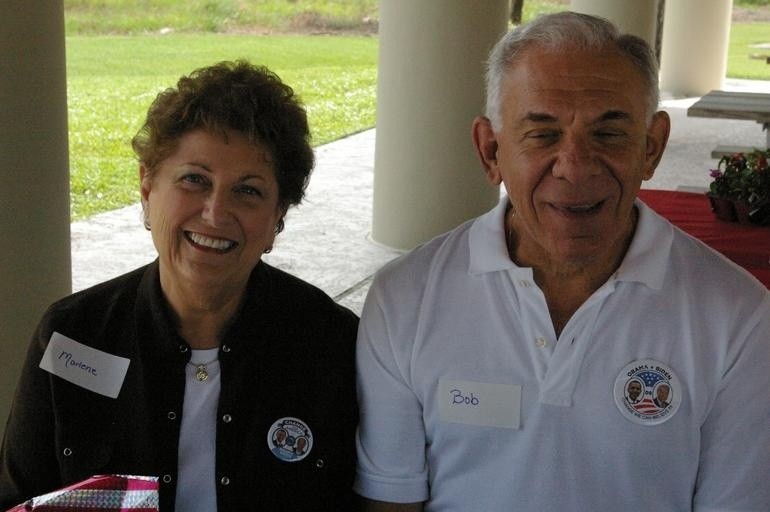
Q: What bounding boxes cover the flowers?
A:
[709,146,770,202]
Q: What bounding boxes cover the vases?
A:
[705,192,770,226]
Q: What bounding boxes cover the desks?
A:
[687,89,770,124]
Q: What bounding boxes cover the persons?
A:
[0,62,359,512]
[351,12,770,512]
[274,430,286,447]
[653,384,670,408]
[626,380,641,404]
[294,438,306,455]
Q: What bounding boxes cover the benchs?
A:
[711,145,765,160]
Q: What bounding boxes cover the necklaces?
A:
[506,210,521,266]
[188,358,219,382]
